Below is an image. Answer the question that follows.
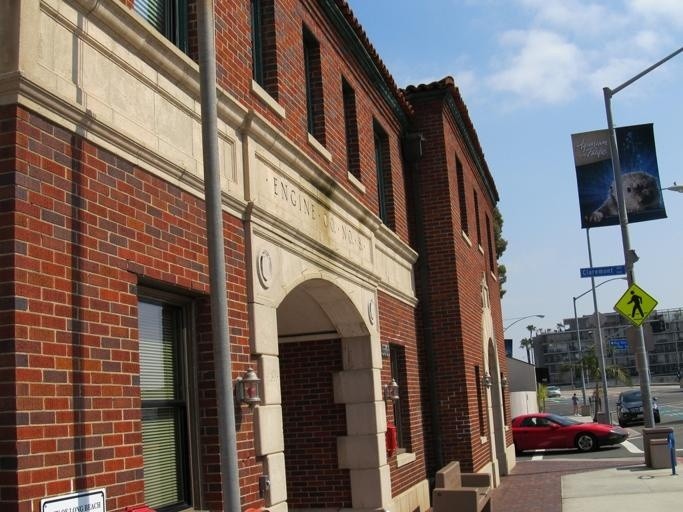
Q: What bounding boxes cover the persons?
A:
[572,393,579,416]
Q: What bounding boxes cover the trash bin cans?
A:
[580,405,591,416]
[597,412,612,424]
[643,427,676,469]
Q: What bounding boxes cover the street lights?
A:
[503,315,544,332]
[574,277,628,405]
[586,185,683,412]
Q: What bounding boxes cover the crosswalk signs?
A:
[613,282,658,328]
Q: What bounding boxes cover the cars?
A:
[546,386,561,398]
[512,413,630,457]
[616,389,660,426]
